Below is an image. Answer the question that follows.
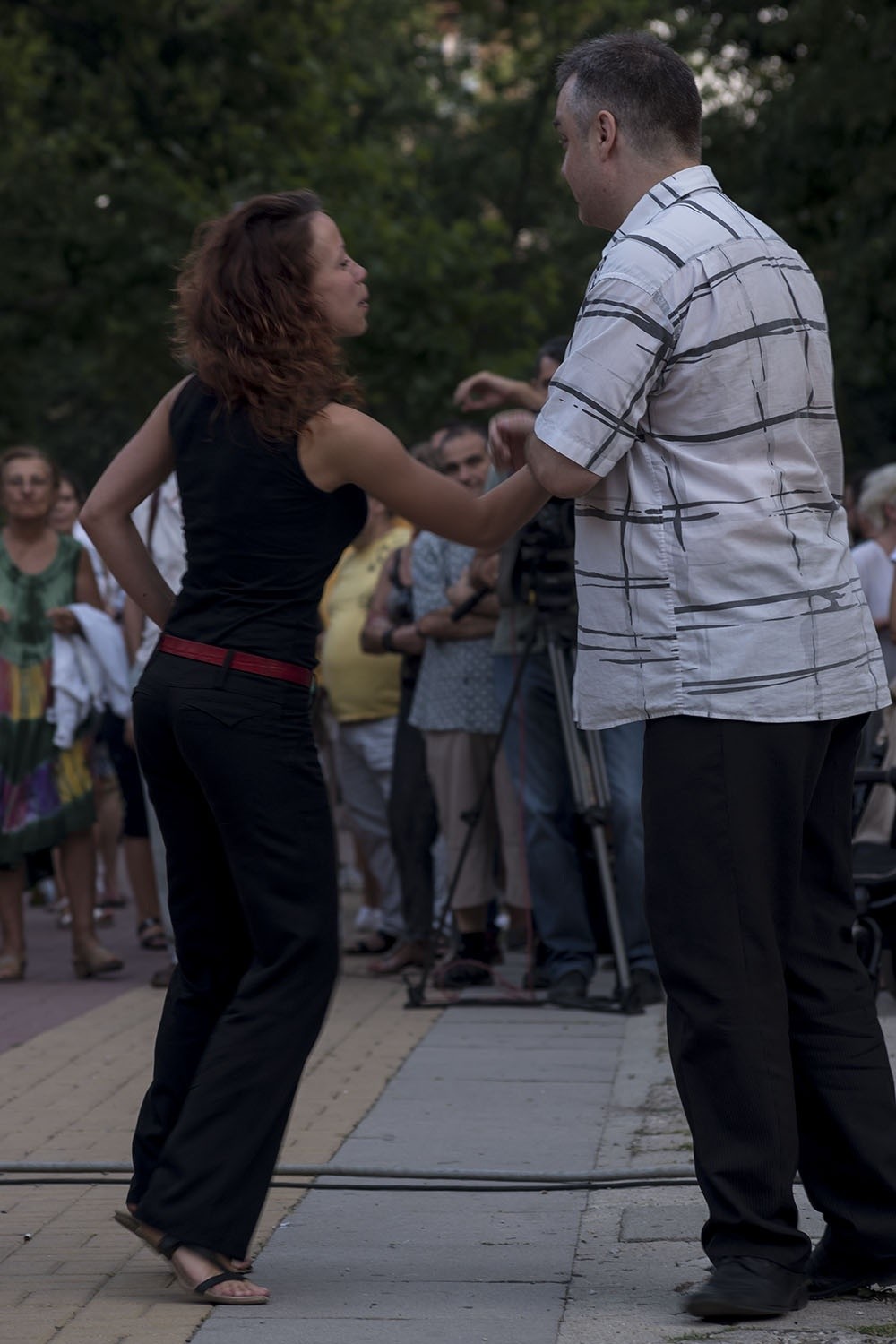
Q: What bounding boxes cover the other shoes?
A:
[152,970,174,988]
[53,896,73,928]
[341,929,399,955]
[809,1234,896,1299]
[522,938,553,990]
[614,968,664,1005]
[367,948,436,975]
[550,971,588,1009]
[686,1257,809,1320]
[92,908,114,927]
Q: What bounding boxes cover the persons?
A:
[0,343,896,1013]
[487,31,896,1321]
[81,190,552,1306]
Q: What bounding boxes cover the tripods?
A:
[407,603,644,1015]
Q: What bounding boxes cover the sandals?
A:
[112,1208,272,1306]
[138,916,169,950]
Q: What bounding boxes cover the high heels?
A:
[72,947,124,979]
[0,954,27,982]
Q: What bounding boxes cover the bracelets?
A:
[380,626,403,654]
